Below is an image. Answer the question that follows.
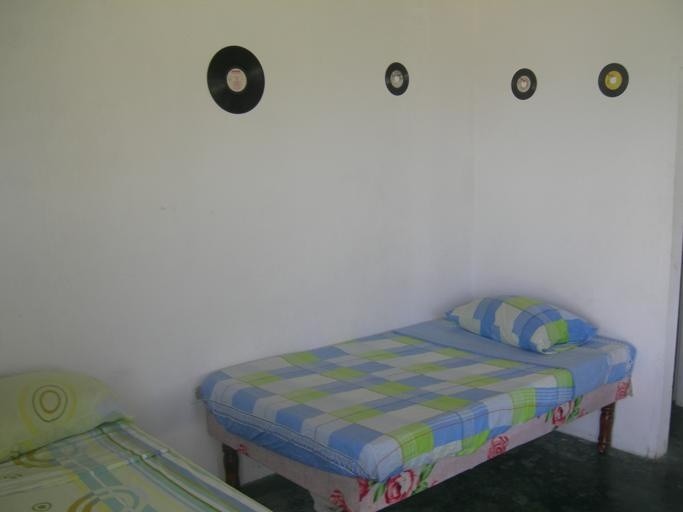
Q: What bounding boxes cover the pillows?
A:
[0,370,127,463]
[441,294,597,354]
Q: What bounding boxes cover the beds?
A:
[0,371,277,512]
[195,315,636,512]
